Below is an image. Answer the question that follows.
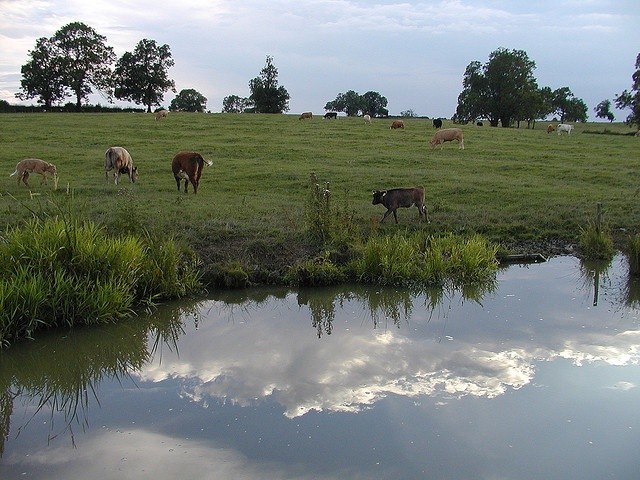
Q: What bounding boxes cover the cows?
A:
[155,110,168,120]
[477,122,483,126]
[428,128,464,150]
[323,112,337,119]
[433,119,442,129]
[104,146,138,185]
[172,151,213,193]
[299,112,312,121]
[547,124,554,134]
[557,124,574,135]
[389,120,404,130]
[372,185,430,226]
[364,115,370,124]
[9,158,57,188]
